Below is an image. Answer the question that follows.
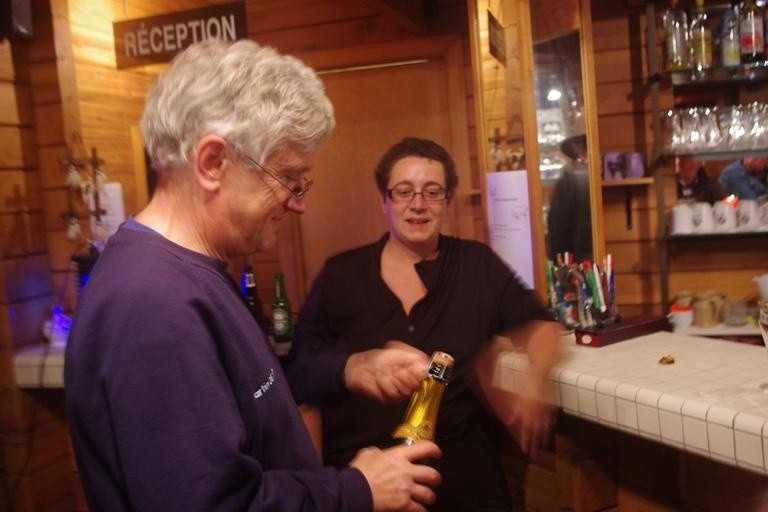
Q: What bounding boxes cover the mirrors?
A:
[530,25,596,271]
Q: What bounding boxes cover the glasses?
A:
[385,185,452,204]
[242,152,314,202]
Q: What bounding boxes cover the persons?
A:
[286,138,564,511]
[539,113,595,264]
[58,35,445,511]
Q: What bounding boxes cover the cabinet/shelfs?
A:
[645,4,768,348]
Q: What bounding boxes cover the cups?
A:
[667,291,754,328]
[670,195,768,234]
[659,102,768,155]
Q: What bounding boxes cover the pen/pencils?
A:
[556,251,622,327]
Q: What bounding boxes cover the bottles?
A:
[240,264,263,329]
[270,271,291,343]
[388,349,456,470]
[662,0,768,71]
